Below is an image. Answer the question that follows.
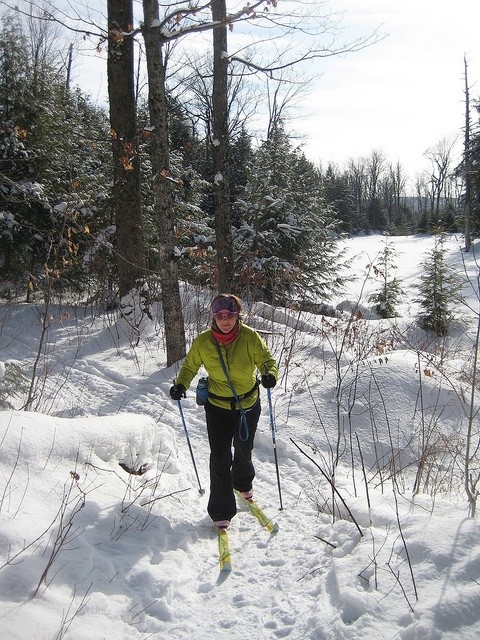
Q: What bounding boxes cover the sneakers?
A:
[215,521,229,528]
[240,489,253,499]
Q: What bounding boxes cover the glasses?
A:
[213,312,238,320]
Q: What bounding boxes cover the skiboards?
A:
[217,489,279,570]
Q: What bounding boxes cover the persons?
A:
[172,294,279,530]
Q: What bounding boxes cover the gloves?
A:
[170,384,187,400]
[262,374,276,388]
